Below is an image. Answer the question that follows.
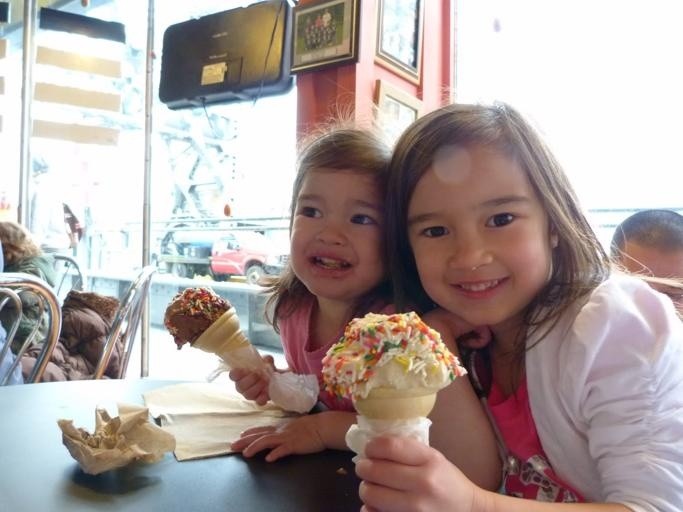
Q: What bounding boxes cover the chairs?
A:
[0,254,158,384]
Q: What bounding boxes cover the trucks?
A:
[152,224,291,285]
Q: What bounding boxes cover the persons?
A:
[348,100,683,510]
[301,8,335,49]
[0,219,59,358]
[228,117,435,465]
[611,210,682,305]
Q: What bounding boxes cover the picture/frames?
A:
[289,1,426,151]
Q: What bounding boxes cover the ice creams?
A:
[163,287,251,368]
[321,311,467,435]
